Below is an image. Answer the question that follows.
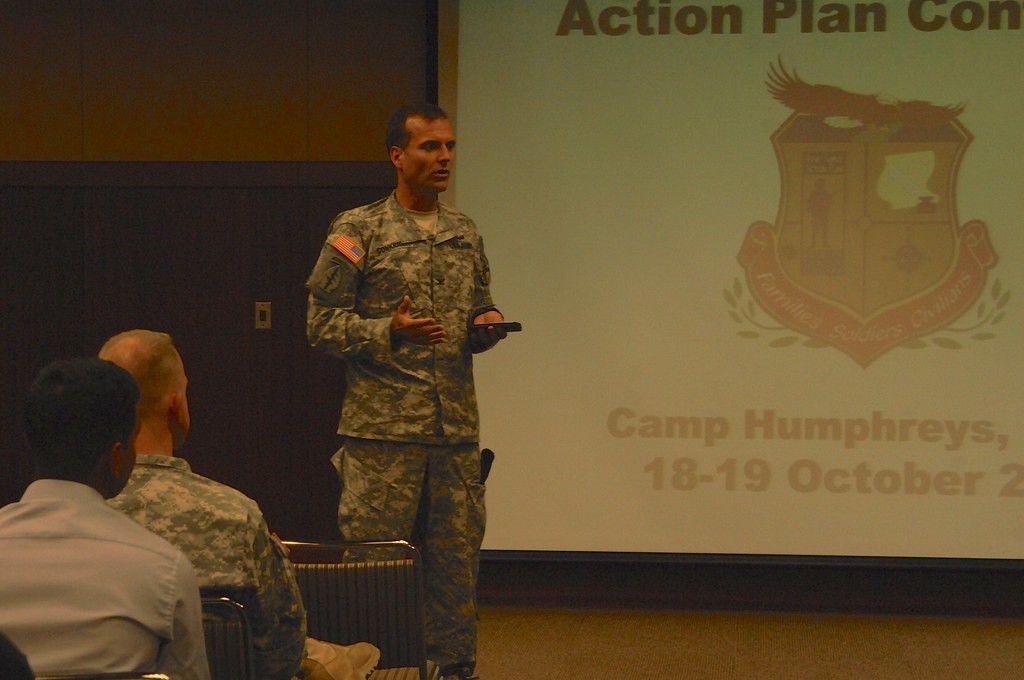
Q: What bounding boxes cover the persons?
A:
[304,100,509,680]
[97,328,381,680]
[0,357,211,680]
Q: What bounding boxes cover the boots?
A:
[296,636,380,679]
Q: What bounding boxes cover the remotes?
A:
[472,322,522,333]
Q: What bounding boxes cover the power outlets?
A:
[255,301,272,329]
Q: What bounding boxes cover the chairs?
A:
[201,596,257,680]
[281,539,444,680]
